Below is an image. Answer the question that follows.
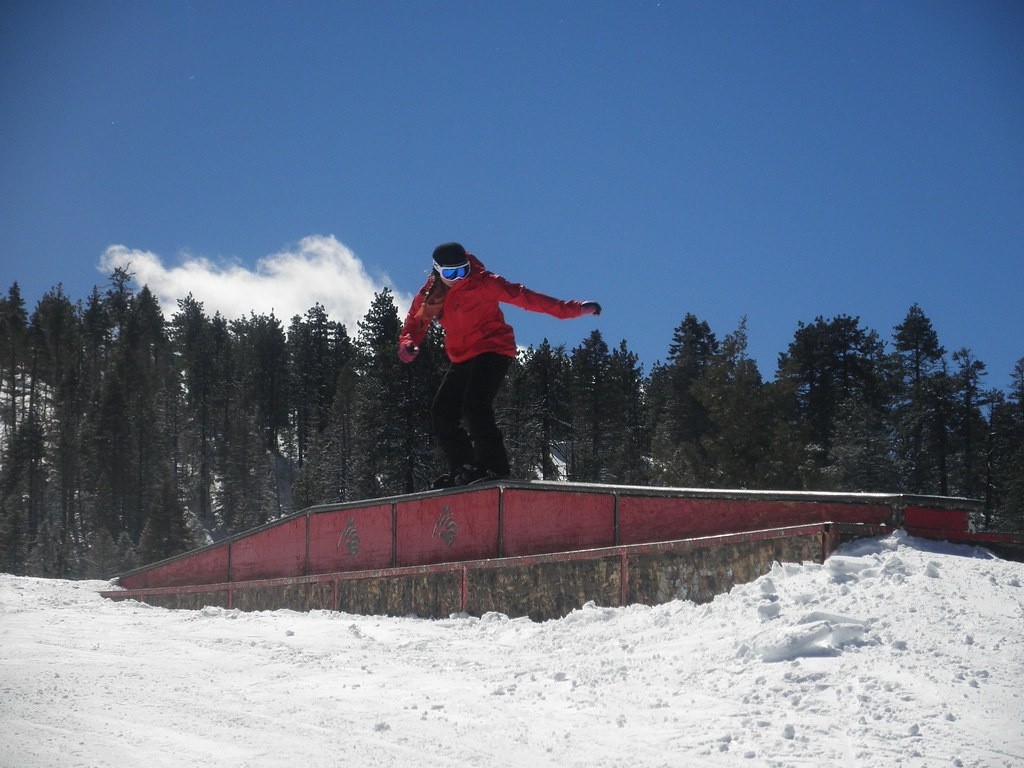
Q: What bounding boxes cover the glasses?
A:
[432,258,472,282]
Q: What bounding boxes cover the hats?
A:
[432,241,467,267]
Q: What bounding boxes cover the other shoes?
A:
[430,465,511,489]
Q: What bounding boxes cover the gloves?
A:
[579,300,603,317]
[397,341,420,364]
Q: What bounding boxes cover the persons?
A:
[398,244,603,492]
